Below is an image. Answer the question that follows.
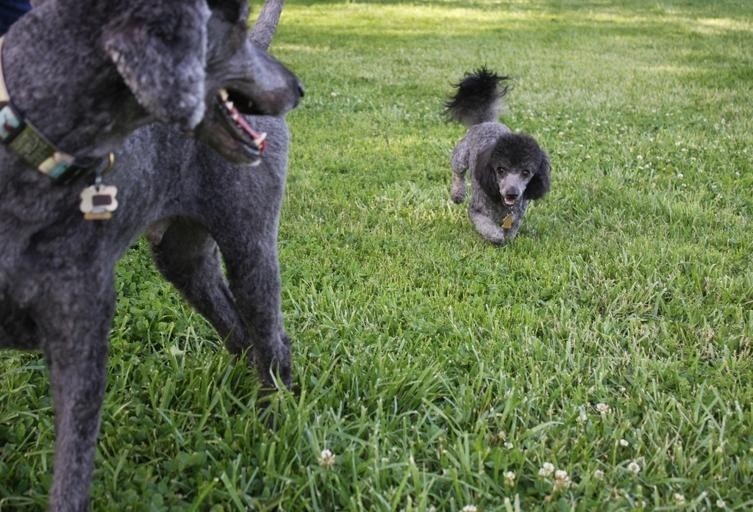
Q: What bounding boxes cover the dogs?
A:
[0,0,306,512]
[437,62,552,246]
